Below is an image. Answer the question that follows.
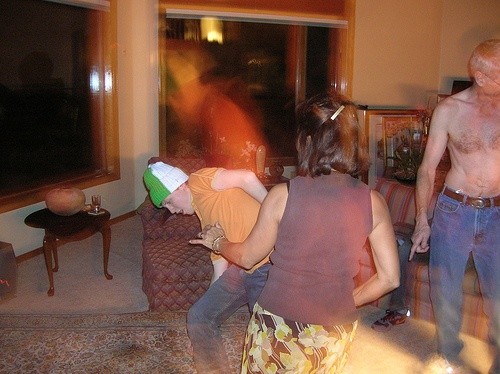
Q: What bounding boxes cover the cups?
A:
[91,195,102,214]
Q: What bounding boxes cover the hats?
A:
[143,161,189,209]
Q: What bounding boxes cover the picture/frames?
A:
[363,108,428,193]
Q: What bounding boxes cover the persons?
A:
[187,93,403,374]
[144,161,276,374]
[369,216,475,333]
[407,38,500,374]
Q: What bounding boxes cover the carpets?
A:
[0,309,253,374]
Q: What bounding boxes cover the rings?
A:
[199,233,203,239]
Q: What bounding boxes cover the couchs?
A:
[141,157,214,312]
[359,177,492,343]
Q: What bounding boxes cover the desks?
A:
[24,206,115,298]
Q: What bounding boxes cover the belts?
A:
[441,186,500,209]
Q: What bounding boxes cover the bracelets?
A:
[212,235,229,255]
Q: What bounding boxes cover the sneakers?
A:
[371,310,409,332]
